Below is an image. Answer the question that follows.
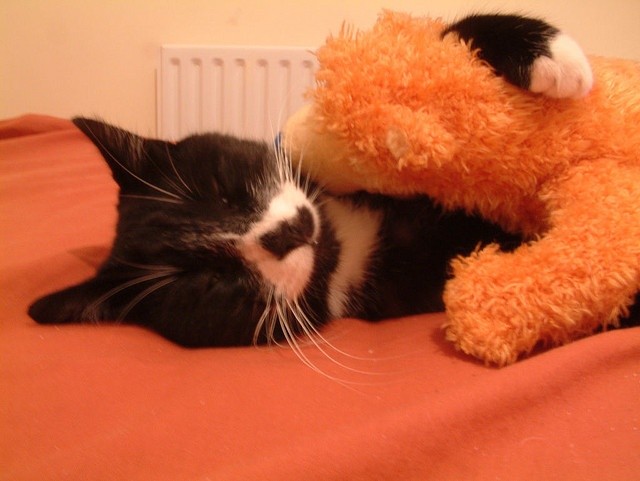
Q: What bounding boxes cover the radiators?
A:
[155,46,325,144]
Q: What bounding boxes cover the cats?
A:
[25,10,594,395]
